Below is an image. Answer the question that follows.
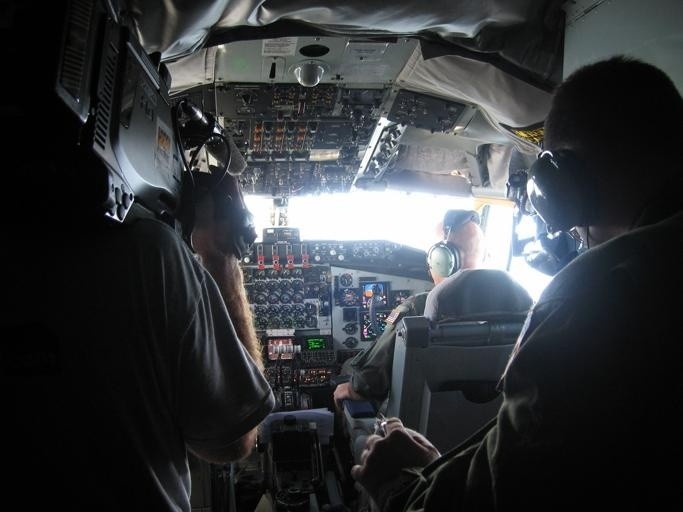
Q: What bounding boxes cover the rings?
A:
[380,417,402,436]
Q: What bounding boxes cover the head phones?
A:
[526,53,602,235]
[425,208,488,277]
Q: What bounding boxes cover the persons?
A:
[0,1,275,510]
[329,216,489,420]
[349,51,681,512]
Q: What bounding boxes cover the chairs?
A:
[332,266,538,475]
